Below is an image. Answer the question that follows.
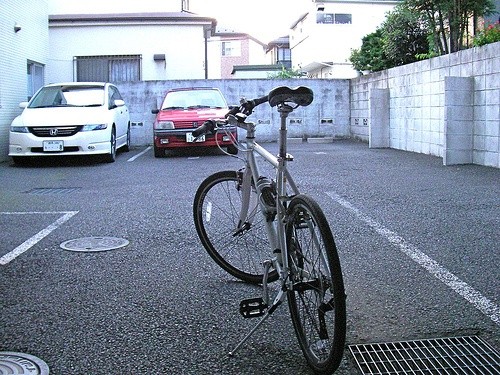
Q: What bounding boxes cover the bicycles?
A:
[192,86,347,375]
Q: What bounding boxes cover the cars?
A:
[151,87,239,159]
[8,82,131,165]
